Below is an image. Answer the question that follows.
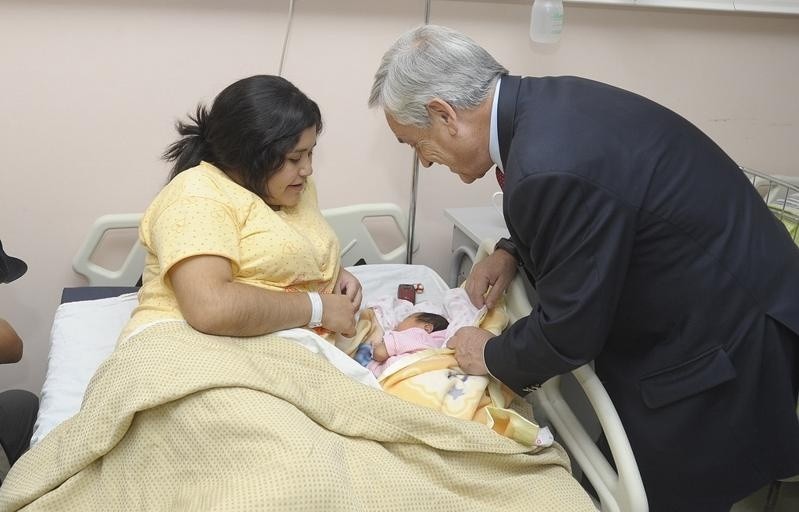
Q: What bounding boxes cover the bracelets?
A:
[308,290,325,331]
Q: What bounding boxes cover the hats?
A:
[0,240,27,284]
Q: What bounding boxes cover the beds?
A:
[24,202,649,511]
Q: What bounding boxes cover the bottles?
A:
[528,0,564,43]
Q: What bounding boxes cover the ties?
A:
[496,166,506,193]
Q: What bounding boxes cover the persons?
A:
[0,240,40,487]
[113,74,364,511]
[368,20,799,510]
[373,311,451,412]
